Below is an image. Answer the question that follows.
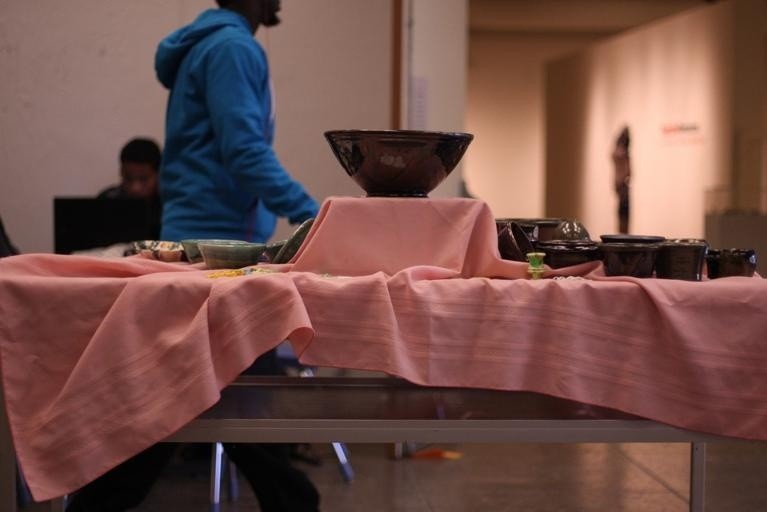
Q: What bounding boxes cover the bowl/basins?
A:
[538,244,598,270]
[599,241,657,277]
[132,238,266,270]
[323,127,476,198]
[656,241,708,281]
[705,248,757,280]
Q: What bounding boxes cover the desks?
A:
[1,246,765,512]
